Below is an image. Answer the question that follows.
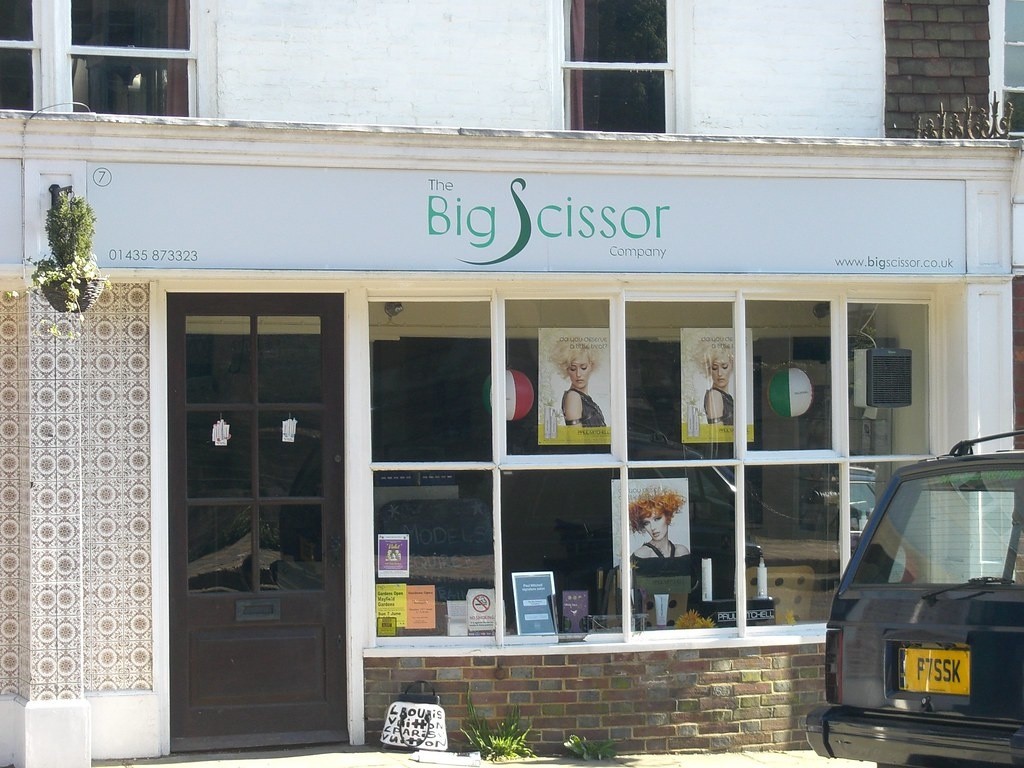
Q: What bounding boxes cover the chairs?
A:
[746,566,815,625]
[606,575,691,628]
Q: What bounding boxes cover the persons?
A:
[688,339,736,426]
[548,337,606,443]
[629,489,690,572]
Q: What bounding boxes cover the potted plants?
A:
[3,193,112,338]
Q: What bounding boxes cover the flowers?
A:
[673,609,716,629]
[785,609,796,624]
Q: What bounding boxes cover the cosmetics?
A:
[757,557,767,599]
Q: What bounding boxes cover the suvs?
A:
[805,429,1024,768]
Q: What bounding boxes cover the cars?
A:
[624,419,878,627]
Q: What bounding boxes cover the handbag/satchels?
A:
[380,679,449,752]
